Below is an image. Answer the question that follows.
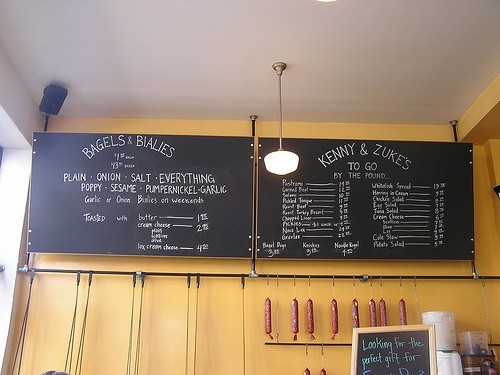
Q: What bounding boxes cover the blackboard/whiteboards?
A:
[351,323,438,375]
[257,137,476,264]
[26,131,256,261]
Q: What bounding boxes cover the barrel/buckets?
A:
[459,331,489,355]
[421,311,457,352]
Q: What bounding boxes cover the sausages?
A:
[263,297,408,375]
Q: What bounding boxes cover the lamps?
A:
[263,62,300,176]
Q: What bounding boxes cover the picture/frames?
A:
[350,323,439,375]
